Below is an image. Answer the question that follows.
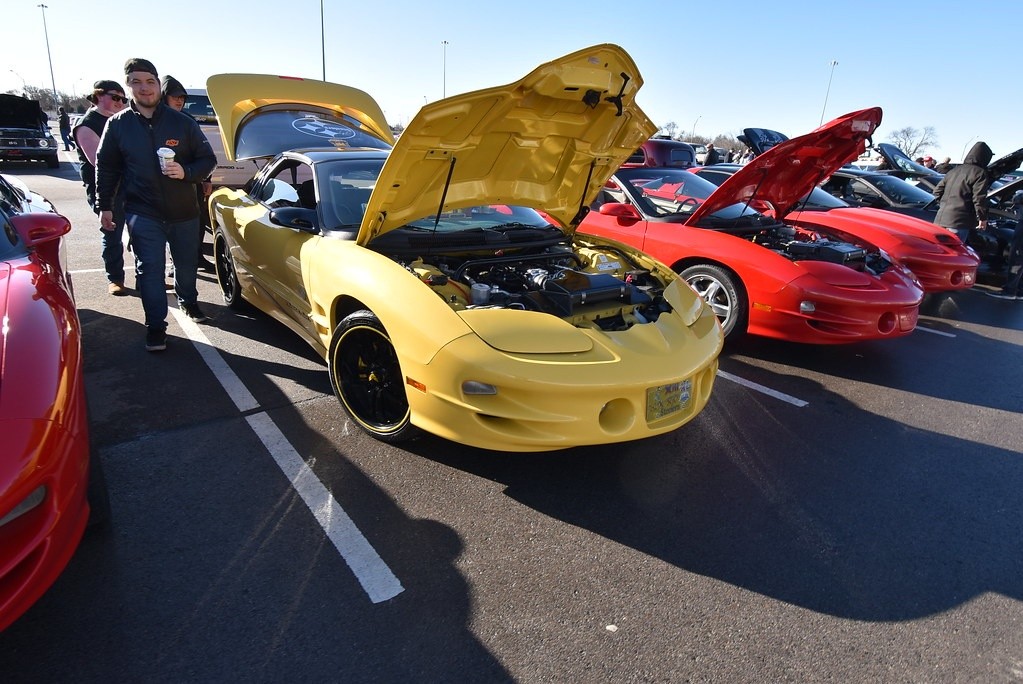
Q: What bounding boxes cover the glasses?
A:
[172,96,186,101]
[104,92,128,104]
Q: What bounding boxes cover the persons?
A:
[724,147,735,163]
[703,143,719,166]
[732,149,756,164]
[73,81,174,294]
[986,192,1023,300]
[95,57,217,350]
[159,75,216,276]
[916,156,953,173]
[40,107,48,125]
[58,107,75,151]
[933,141,994,241]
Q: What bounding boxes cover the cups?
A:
[157,147,175,174]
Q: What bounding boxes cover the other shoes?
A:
[72,145,75,150]
[62,148,70,151]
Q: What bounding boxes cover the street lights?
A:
[441,41,451,99]
[37,3,60,116]
[820,61,839,126]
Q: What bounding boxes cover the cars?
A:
[0,93,61,168]
[637,163,981,293]
[491,107,926,342]
[0,174,91,631]
[184,88,313,185]
[615,125,1023,265]
[207,41,724,452]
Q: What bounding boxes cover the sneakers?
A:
[107,279,125,296]
[198,255,216,274]
[164,283,174,289]
[984,289,1016,300]
[180,300,206,323]
[169,264,174,277]
[146,329,168,351]
[1016,290,1023,301]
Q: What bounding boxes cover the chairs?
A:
[298,180,342,210]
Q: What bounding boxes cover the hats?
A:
[922,156,933,161]
[167,86,189,98]
[86,80,125,105]
[125,58,158,78]
